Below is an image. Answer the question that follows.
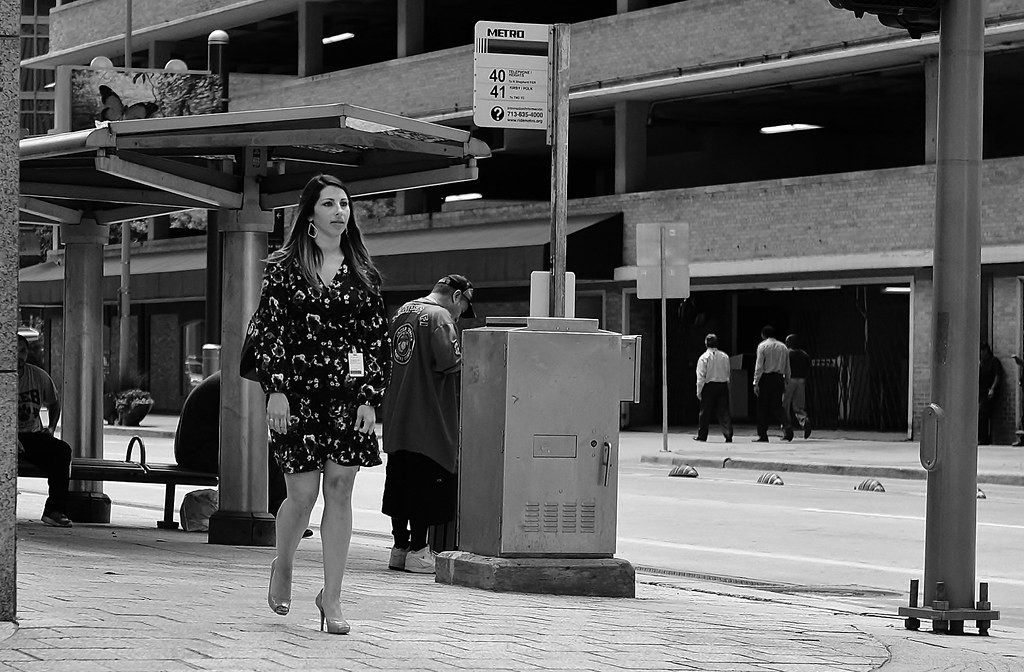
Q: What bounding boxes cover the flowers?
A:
[115,389,155,412]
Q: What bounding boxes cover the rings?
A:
[269,417,276,422]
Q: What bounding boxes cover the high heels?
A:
[315,588,350,633]
[268,558,293,615]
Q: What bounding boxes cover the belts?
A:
[791,375,806,378]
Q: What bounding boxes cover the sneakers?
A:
[388,541,412,571]
[404,543,440,574]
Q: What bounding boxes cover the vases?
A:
[118,404,154,427]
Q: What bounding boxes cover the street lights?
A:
[88,56,189,391]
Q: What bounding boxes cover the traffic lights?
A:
[876,0,942,40]
[829,0,903,19]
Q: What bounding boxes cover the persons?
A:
[752,326,794,441]
[693,334,733,442]
[978,342,1005,445]
[18,334,74,528]
[782,334,811,439]
[175,370,313,538]
[382,274,477,573]
[1011,356,1024,446]
[240,174,393,634]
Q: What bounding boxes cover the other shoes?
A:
[41,498,73,528]
[800,417,812,439]
[693,437,706,442]
[980,439,992,445]
[752,435,769,443]
[726,437,732,442]
[302,530,313,538]
[780,424,794,442]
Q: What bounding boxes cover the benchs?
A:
[18,435,218,530]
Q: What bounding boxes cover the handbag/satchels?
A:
[179,488,218,532]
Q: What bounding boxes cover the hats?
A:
[437,274,478,320]
[705,333,720,347]
